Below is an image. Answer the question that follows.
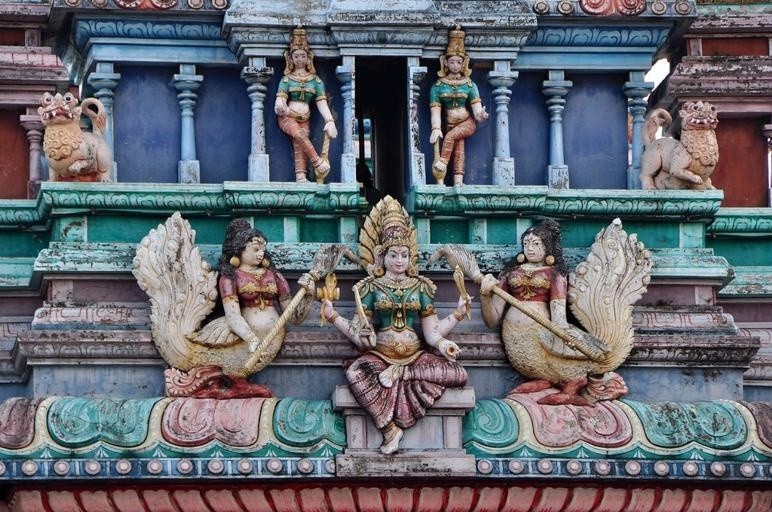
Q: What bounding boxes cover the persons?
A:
[322,195,471,455]
[219,220,316,362]
[429,30,488,186]
[274,27,338,185]
[479,220,578,348]
